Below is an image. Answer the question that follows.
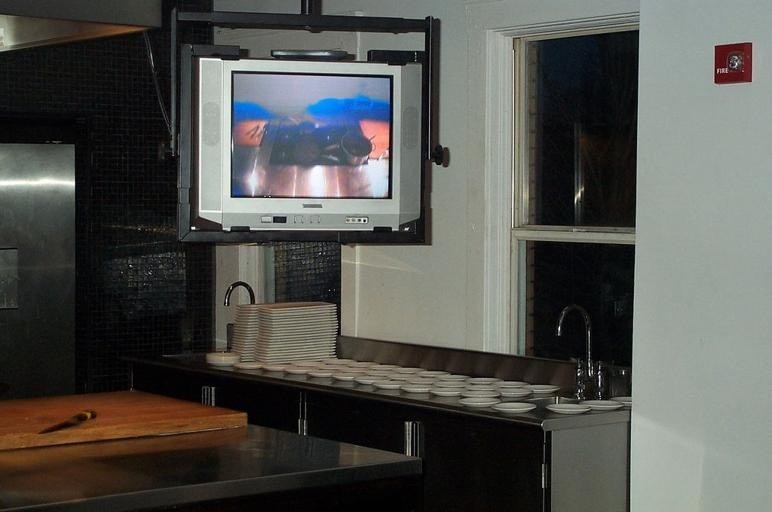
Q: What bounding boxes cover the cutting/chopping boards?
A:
[0,391,247,452]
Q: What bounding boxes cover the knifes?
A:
[40,408,97,435]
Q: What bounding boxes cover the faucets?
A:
[224,281,255,305]
[554,304,594,375]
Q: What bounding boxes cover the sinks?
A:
[162,351,228,359]
[526,396,580,410]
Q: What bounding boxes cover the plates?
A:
[207,351,632,414]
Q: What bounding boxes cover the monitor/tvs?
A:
[191,56,424,234]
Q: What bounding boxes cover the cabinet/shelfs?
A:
[131,364,301,436]
[304,392,541,511]
